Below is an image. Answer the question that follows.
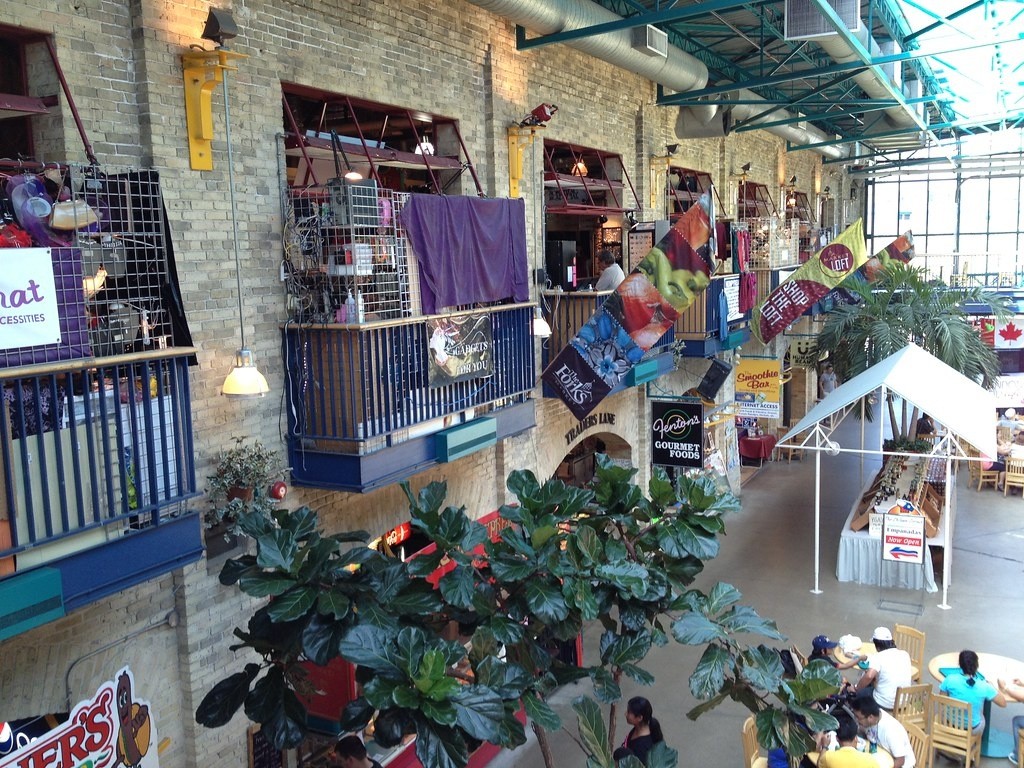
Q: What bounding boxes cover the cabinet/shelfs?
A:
[282,133,464,436]
[1,92,176,365]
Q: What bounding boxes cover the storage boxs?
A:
[850,461,942,537]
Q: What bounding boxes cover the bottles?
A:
[345,288,366,324]
[870,737,877,754]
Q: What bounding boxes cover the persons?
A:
[621,696,663,766]
[981,440,1012,491]
[334,736,382,768]
[847,626,911,711]
[939,650,1007,761]
[767,701,818,768]
[916,412,937,438]
[997,429,1005,460]
[613,747,634,768]
[594,250,625,292]
[819,366,838,398]
[817,715,879,768]
[808,635,868,698]
[852,695,916,768]
[997,678,1024,765]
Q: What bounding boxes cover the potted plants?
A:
[202,436,288,544]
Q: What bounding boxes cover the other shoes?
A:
[1008,753,1018,765]
[1012,488,1017,495]
[998,482,1004,492]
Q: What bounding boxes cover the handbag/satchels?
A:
[48,168,98,230]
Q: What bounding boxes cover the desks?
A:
[834,642,878,670]
[928,650,1024,759]
[737,429,776,470]
[835,468,958,594]
[806,731,894,765]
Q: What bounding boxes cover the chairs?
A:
[777,419,807,465]
[741,624,1024,768]
[957,412,1024,499]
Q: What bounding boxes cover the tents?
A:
[775,343,997,611]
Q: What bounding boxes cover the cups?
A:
[755,392,766,404]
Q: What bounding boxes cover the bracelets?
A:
[1003,687,1007,692]
[822,745,827,749]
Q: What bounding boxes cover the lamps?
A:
[816,186,831,223]
[215,46,271,401]
[415,121,435,156]
[508,103,559,198]
[182,7,251,172]
[704,409,721,428]
[626,210,639,229]
[528,131,553,340]
[780,176,800,211]
[729,162,754,214]
[332,129,362,180]
[731,346,742,366]
[599,216,608,223]
[650,143,683,210]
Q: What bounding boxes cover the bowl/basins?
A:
[856,737,866,751]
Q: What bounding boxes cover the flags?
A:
[747,217,869,347]
[541,184,718,422]
[818,230,916,312]
[979,319,1024,349]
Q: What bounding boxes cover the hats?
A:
[870,626,893,641]
[812,635,837,650]
